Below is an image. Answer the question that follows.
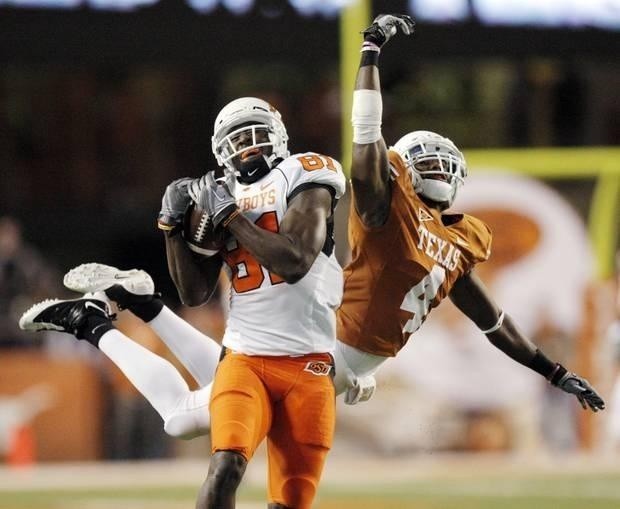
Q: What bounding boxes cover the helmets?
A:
[211,96,289,177]
[389,130,467,207]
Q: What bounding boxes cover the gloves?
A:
[363,14,415,45]
[157,170,240,231]
[546,362,606,413]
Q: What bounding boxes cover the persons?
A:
[19,13,605,509]
[160,96,348,509]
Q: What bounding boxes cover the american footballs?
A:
[182,181,231,262]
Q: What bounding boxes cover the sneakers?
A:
[19,262,155,340]
